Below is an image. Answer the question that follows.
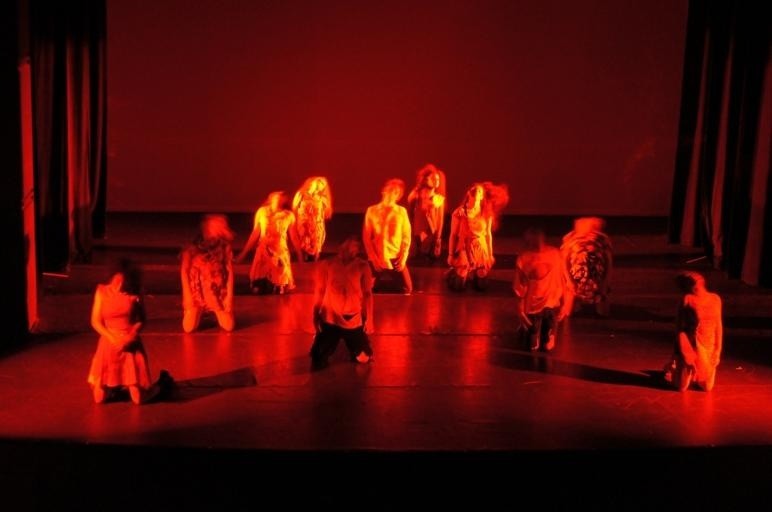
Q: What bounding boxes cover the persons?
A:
[407,162,447,262]
[306,235,377,373]
[663,268,727,395]
[236,189,298,296]
[291,174,335,271]
[178,211,237,333]
[444,181,514,293]
[84,262,176,407]
[558,216,614,318]
[359,177,414,296]
[512,223,575,354]
